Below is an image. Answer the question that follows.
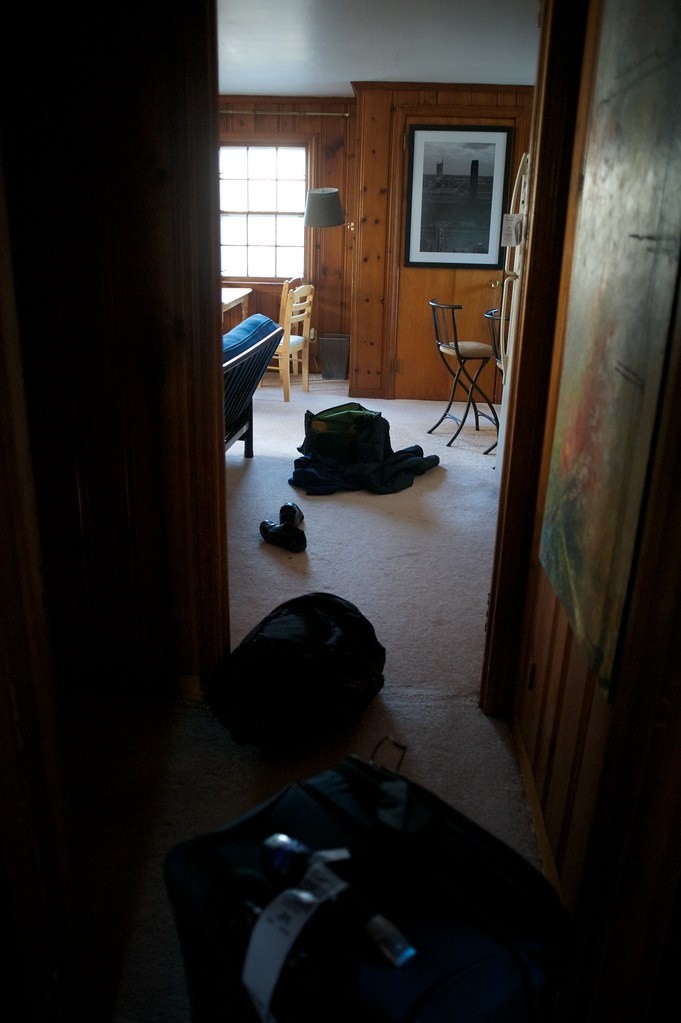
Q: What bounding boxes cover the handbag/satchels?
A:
[297,402,394,462]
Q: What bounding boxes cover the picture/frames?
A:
[403,123,514,270]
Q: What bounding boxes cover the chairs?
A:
[258,284,315,402]
[222,313,285,458]
[484,308,510,378]
[277,278,302,377]
[428,297,500,456]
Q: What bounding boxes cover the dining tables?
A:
[222,287,252,323]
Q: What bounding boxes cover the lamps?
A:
[303,187,354,231]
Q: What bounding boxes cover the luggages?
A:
[160,752,564,1023]
